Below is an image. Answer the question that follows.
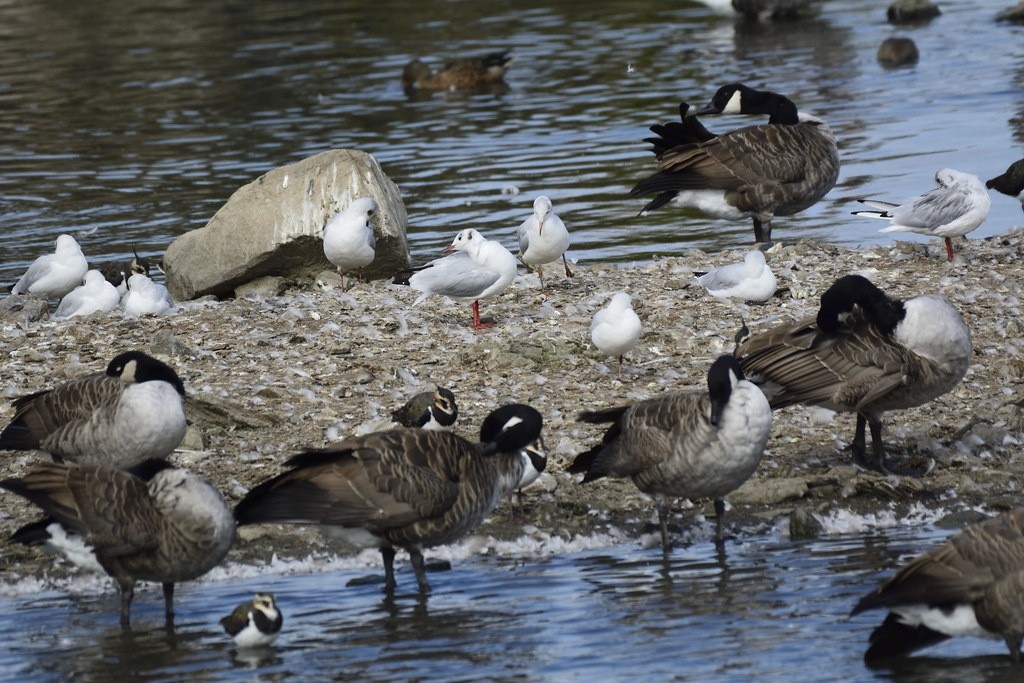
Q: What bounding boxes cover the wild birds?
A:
[848,507,1024,667]
[324,197,378,291]
[391,228,517,329]
[111,243,169,317]
[516,195,573,287]
[11,225,99,318]
[218,592,283,646]
[345,384,458,437]
[696,249,777,306]
[588,292,642,379]
[733,274,972,456]
[231,403,547,593]
[400,46,1024,262]
[47,270,120,321]
[0,351,187,477]
[564,355,771,546]
[0,455,236,626]
